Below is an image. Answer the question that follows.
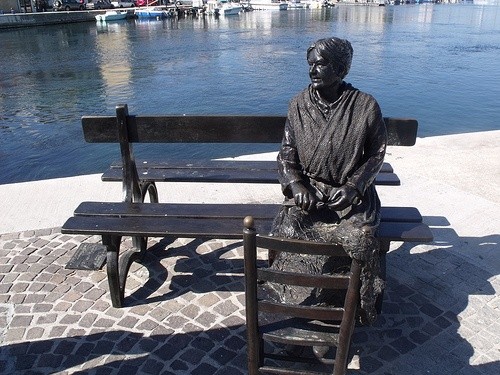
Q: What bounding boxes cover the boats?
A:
[95,0,336,23]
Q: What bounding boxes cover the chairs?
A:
[244,215,374,375]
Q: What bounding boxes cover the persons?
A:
[264,38,388,358]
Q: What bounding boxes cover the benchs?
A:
[62,103,434,308]
